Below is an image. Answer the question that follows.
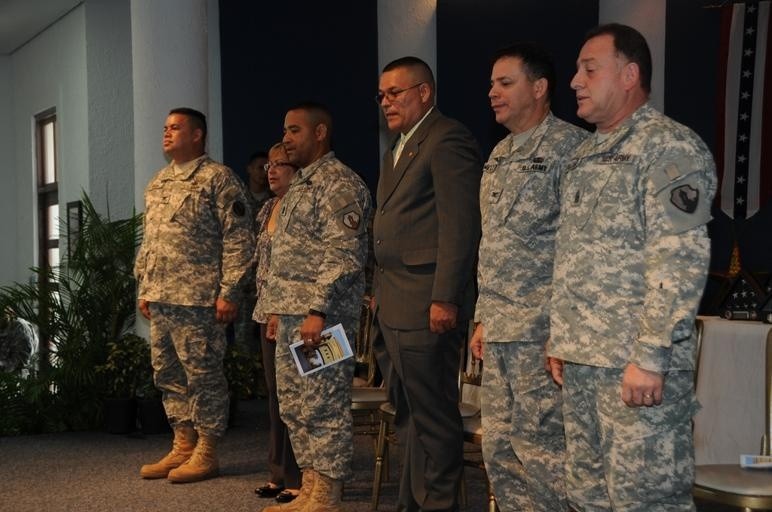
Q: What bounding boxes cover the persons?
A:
[133,108,255,482]
[256,141,303,502]
[543,26,714,512]
[267,104,371,511]
[471,50,596,512]
[371,58,482,512]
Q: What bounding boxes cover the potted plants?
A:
[0,205,139,435]
[104,332,162,435]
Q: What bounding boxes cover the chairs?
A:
[694,328,772,512]
[692,321,704,445]
[456,410,499,512]
[357,296,372,369]
[350,358,391,491]
[373,325,483,512]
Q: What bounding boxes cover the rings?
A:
[307,337,313,342]
[644,393,654,398]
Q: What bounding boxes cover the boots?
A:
[169,431,225,481]
[279,473,343,512]
[139,428,198,478]
[262,469,318,512]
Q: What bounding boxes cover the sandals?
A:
[276,488,299,502]
[255,482,284,497]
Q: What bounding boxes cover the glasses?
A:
[264,162,294,171]
[375,81,424,104]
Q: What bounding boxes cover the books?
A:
[289,324,354,379]
